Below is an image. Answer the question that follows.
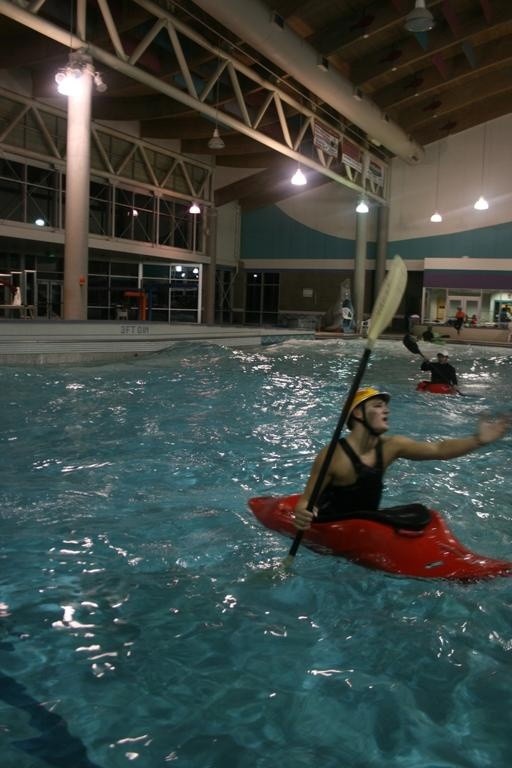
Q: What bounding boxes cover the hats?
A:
[436,349,450,357]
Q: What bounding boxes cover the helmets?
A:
[342,385,393,430]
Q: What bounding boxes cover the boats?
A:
[416,380,457,394]
[245,492,512,582]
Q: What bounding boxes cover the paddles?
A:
[433,334,450,338]
[284,254,408,566]
[403,335,463,396]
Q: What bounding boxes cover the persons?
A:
[292,383,511,532]
[421,324,436,344]
[469,314,478,327]
[454,306,466,325]
[420,350,459,390]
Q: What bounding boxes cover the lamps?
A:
[474,122,490,210]
[54,45,109,95]
[430,133,444,224]
[178,78,225,216]
[354,140,370,215]
[291,93,309,189]
[403,2,436,31]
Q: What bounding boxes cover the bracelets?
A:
[473,432,490,448]
